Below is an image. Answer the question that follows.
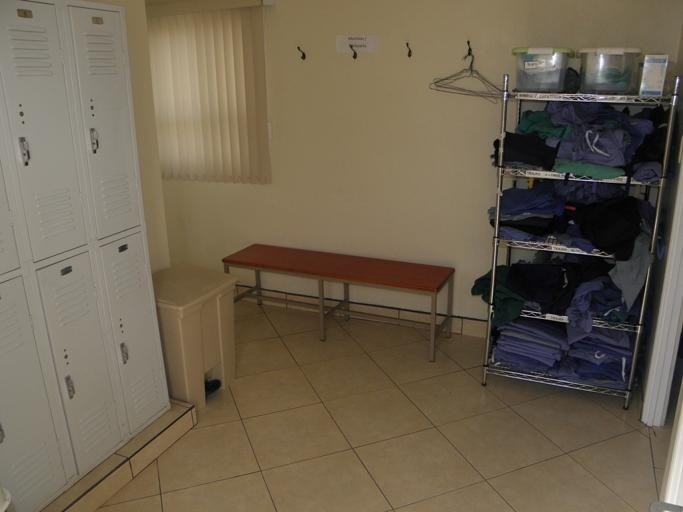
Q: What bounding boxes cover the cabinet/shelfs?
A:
[0,0,170,512]
[482,75,681,407]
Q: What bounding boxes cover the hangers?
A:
[429,54,506,103]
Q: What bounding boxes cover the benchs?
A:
[222,243,455,362]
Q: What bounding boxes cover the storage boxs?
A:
[512,45,575,93]
[576,47,643,93]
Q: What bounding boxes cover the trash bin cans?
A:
[151,265,239,410]
